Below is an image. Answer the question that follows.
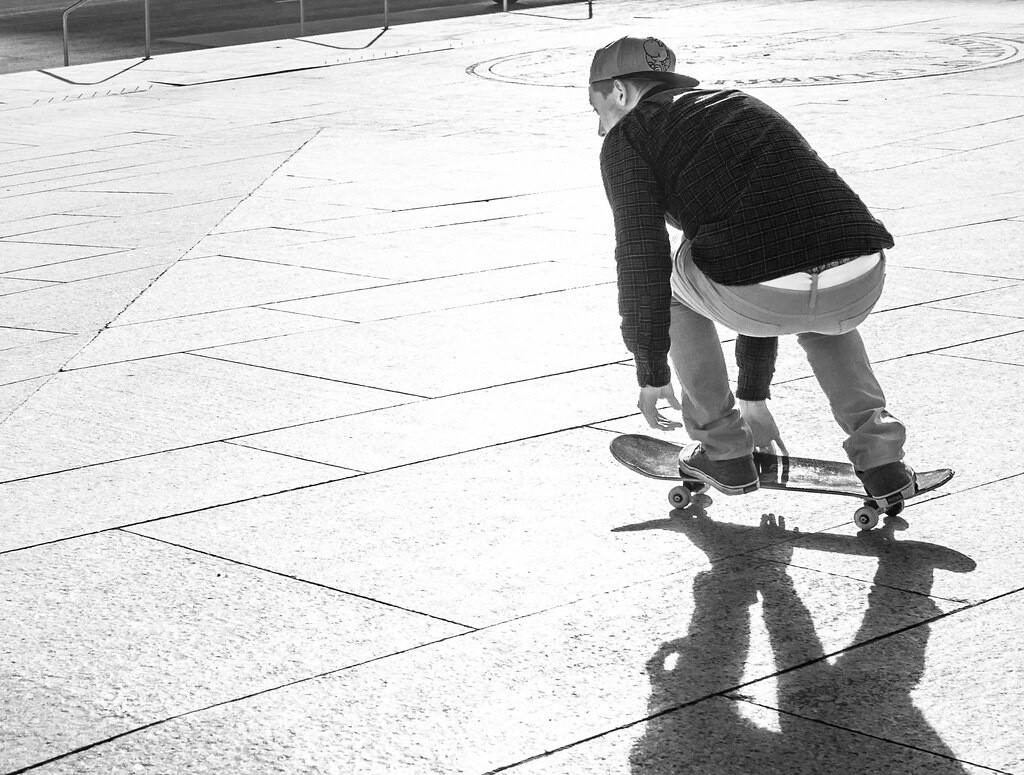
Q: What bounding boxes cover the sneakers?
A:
[678,443,760,496]
[855,460,917,509]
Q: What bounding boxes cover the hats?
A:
[589,35,700,88]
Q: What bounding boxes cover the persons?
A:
[589,35,918,509]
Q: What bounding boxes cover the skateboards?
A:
[609,433,957,529]
